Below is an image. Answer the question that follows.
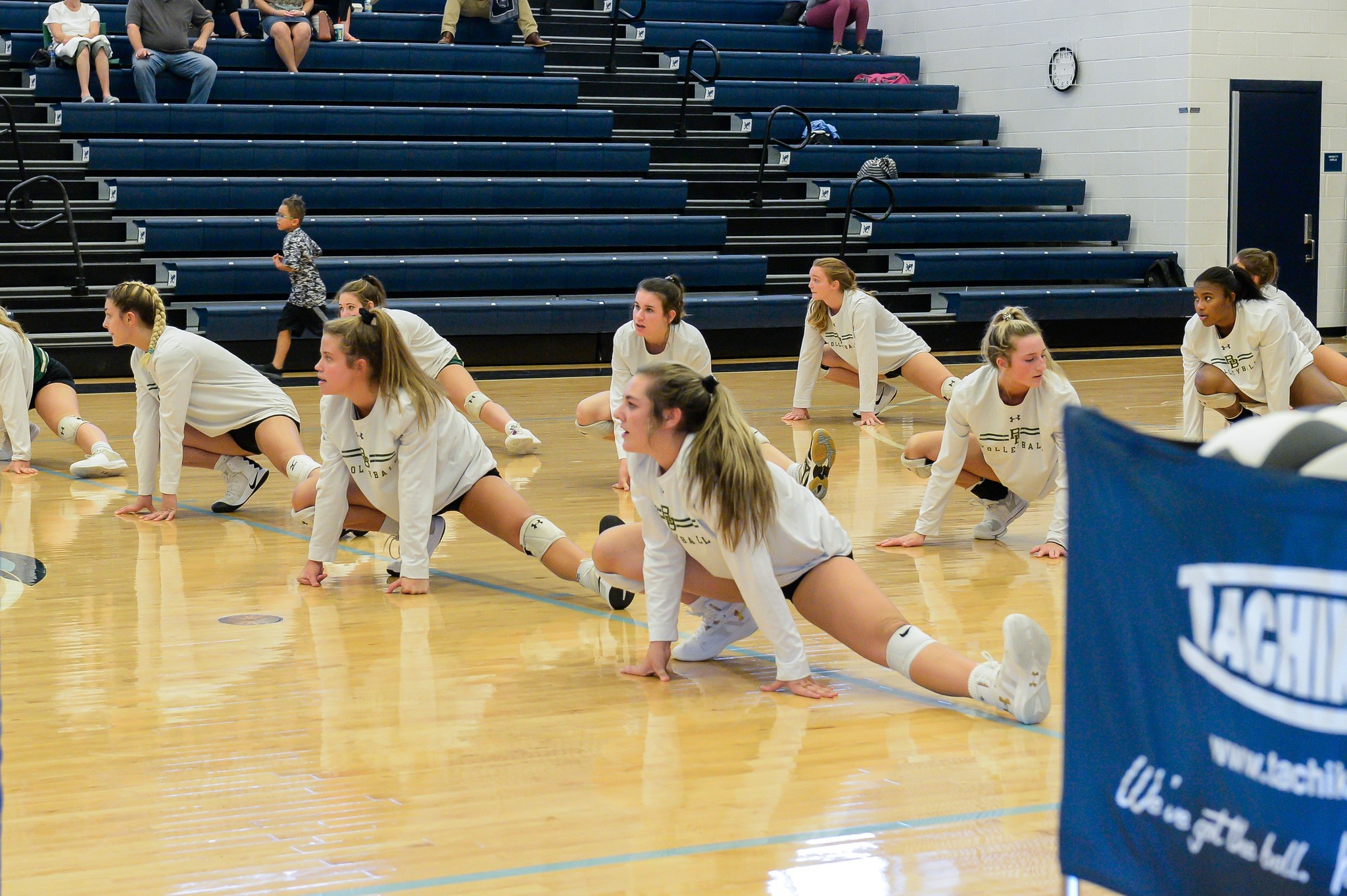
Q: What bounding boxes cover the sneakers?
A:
[979,613,1051,725]
[504,422,542,455]
[968,487,1030,540]
[852,379,898,419]
[670,597,760,662]
[339,528,369,541]
[830,43,853,55]
[69,447,129,480]
[0,420,41,460]
[250,363,284,380]
[795,428,836,501]
[383,515,447,576]
[579,515,636,611]
[857,43,871,55]
[210,455,270,513]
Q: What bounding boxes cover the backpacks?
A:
[807,129,843,145]
[1144,257,1189,289]
[857,154,899,181]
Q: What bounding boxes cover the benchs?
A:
[1,1,1196,344]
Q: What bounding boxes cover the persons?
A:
[330,275,543,455]
[803,0,872,55]
[194,0,361,73]
[101,279,369,539]
[591,362,1051,725]
[248,194,332,383]
[574,274,836,502]
[0,304,128,478]
[875,306,1081,559]
[1183,265,1347,444]
[1230,246,1347,389]
[781,257,962,427]
[43,0,119,104]
[437,0,552,48]
[296,305,639,610]
[126,0,218,104]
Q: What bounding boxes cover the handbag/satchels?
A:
[852,72,910,84]
[27,49,66,70]
[311,9,335,42]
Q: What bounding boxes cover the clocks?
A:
[1048,48,1079,92]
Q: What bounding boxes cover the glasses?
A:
[275,212,294,220]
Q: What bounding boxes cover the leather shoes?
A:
[524,32,552,47]
[438,30,454,44]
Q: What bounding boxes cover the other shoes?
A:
[102,95,120,103]
[208,31,219,39]
[81,94,95,103]
[236,29,252,39]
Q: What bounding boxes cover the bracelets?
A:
[301,10,306,16]
[272,10,277,15]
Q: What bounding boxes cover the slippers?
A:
[344,36,360,41]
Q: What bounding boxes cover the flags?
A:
[1059,404,1347,896]
